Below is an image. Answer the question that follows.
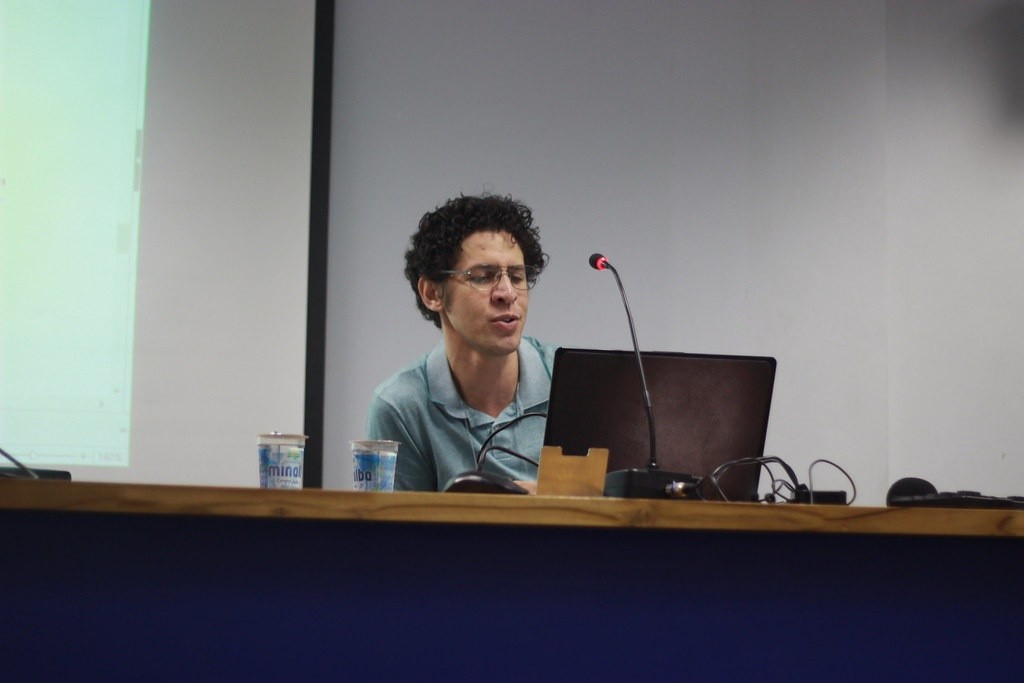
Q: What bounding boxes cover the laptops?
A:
[544,347,778,499]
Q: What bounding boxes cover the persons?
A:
[367,194,554,494]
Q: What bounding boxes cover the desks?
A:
[0,473,1024,683]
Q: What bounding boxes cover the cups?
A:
[260,434,308,490]
[349,439,401,492]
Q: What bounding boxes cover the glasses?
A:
[432,264,538,291]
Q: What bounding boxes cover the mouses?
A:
[441,469,527,494]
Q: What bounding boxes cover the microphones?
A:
[589,252,704,501]
